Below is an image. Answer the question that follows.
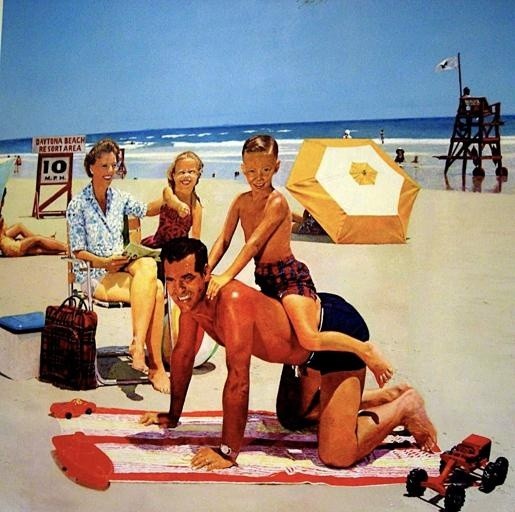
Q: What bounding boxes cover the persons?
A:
[0,218,67,257]
[141,149,203,239]
[14,155,22,173]
[393,146,406,163]
[138,236,438,472]
[5,221,56,240]
[459,84,474,98]
[292,207,322,237]
[379,129,386,144]
[205,135,394,389]
[65,138,171,396]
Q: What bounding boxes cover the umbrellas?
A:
[0,159,16,211]
[286,136,420,246]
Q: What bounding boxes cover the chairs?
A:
[62,214,174,384]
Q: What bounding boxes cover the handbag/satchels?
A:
[39,295,98,390]
